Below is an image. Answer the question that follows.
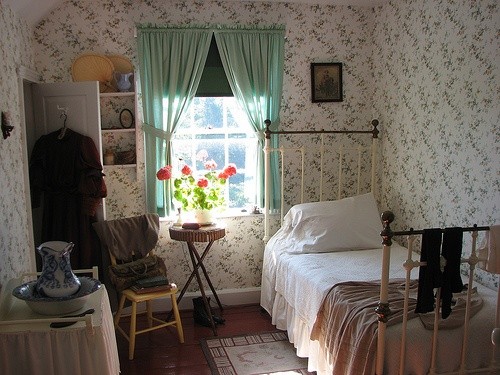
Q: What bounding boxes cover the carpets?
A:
[201,330,319,375]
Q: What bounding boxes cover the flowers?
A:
[156,149,237,212]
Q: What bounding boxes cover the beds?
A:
[264,119,500,375]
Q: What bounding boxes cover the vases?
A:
[183,208,214,225]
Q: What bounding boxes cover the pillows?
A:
[282,193,385,254]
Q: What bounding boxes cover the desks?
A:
[170,225,225,336]
[1,285,122,375]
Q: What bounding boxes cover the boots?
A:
[198,296,226,323]
[192,298,217,326]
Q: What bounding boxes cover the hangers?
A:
[57,114,70,132]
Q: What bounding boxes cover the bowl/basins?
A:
[14,277,102,316]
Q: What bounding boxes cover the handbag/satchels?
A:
[112,254,167,291]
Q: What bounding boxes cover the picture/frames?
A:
[311,61,343,103]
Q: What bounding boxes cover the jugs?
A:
[114,71,133,93]
[33,240,80,297]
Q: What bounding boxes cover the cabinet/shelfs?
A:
[102,92,137,167]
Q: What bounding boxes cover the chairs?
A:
[93,212,185,359]
